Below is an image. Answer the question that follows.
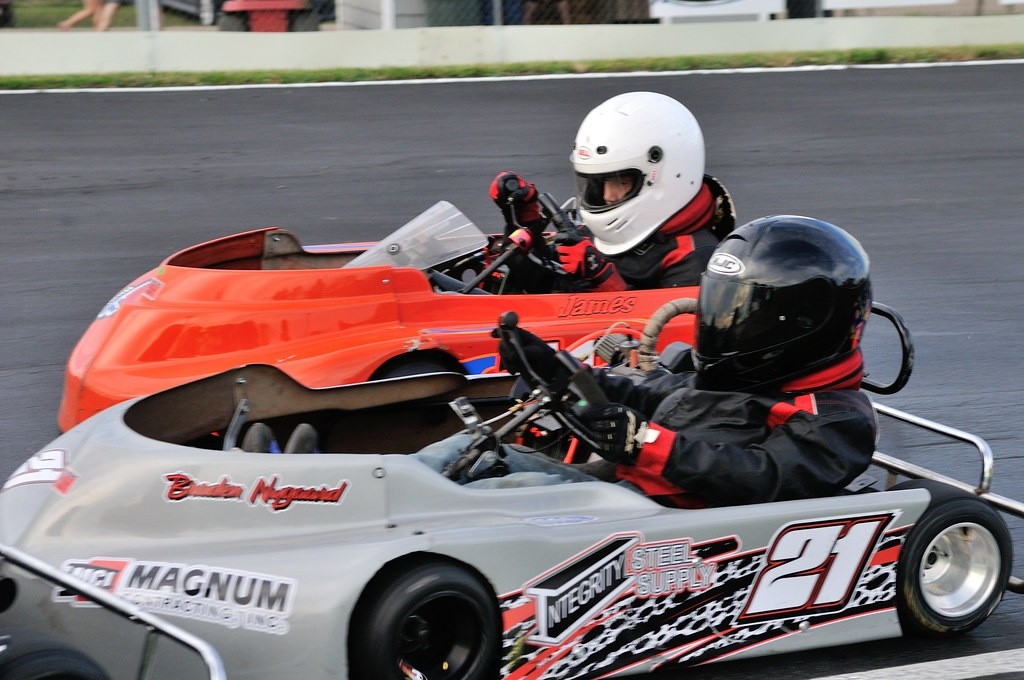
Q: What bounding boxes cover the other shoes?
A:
[283,423,321,454]
[241,422,281,454]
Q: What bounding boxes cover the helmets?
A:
[570,91,705,256]
[693,213,871,393]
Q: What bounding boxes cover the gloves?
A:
[550,402,650,467]
[553,232,617,279]
[488,170,538,212]
[491,325,556,376]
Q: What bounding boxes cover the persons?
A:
[58,0,118,33]
[439,92,721,296]
[240,214,876,508]
